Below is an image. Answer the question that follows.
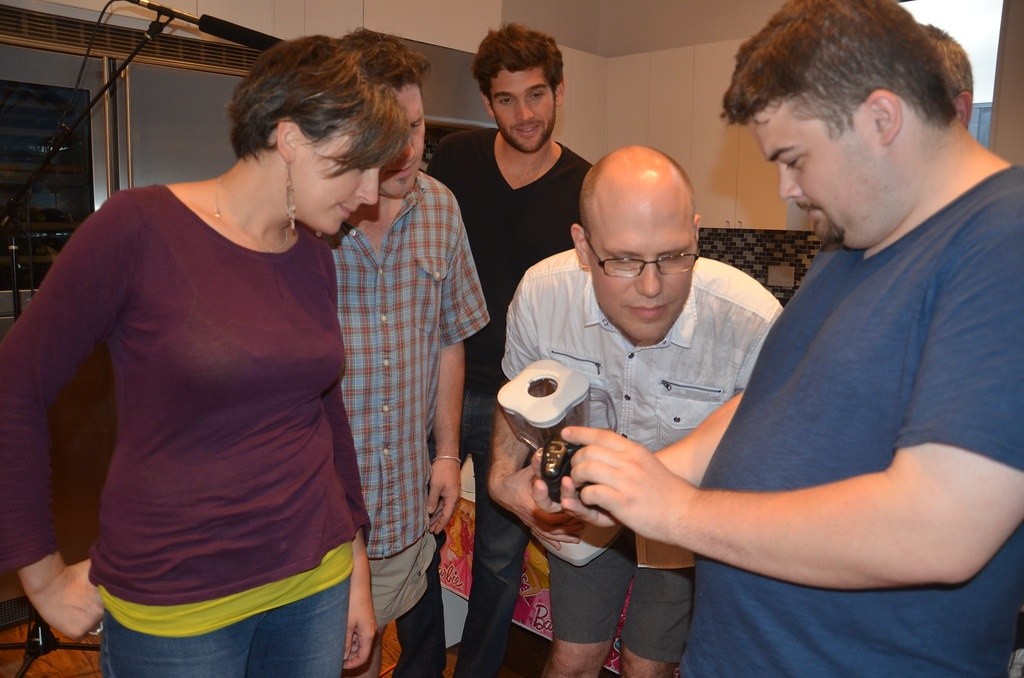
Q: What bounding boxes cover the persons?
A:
[393,23,592,678]
[326,29,492,678]
[0,33,406,678]
[488,146,786,678]
[532,0,1024,678]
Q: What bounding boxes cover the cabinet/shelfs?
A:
[557,38,815,231]
[52,0,503,56]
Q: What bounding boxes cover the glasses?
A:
[584,235,701,278]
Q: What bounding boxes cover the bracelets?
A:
[433,456,461,463]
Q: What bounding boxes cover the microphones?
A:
[127,0,288,51]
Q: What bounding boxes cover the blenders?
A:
[500,358,624,567]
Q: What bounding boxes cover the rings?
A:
[89,620,104,636]
